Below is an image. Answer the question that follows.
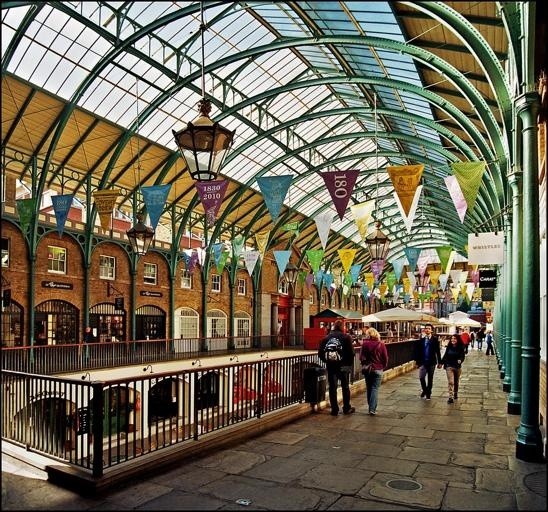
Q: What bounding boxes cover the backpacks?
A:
[324,333,348,363]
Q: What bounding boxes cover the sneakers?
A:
[448,398,454,403]
[421,390,426,397]
[426,393,431,400]
[330,409,339,417]
[344,407,355,415]
[369,411,377,415]
[454,392,457,399]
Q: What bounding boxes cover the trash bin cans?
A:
[304,366,327,414]
[476,332,479,341]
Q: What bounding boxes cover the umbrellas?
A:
[364,303,482,340]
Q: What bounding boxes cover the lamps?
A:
[126,77,156,257]
[172,1,236,181]
[364,92,391,261]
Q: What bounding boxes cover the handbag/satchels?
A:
[361,360,371,376]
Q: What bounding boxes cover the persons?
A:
[321,323,363,346]
[319,319,356,416]
[357,327,389,415]
[415,323,443,401]
[485,330,495,355]
[477,329,484,349]
[437,334,465,403]
[469,330,476,347]
